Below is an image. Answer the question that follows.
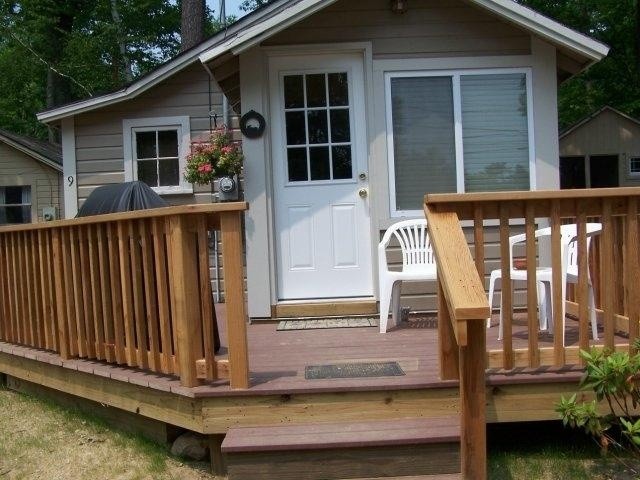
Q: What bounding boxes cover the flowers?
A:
[183,125,243,186]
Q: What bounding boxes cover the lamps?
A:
[390,1,409,15]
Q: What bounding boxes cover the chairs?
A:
[487,222,604,342]
[378,216,439,334]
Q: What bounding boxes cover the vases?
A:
[219,177,240,202]
